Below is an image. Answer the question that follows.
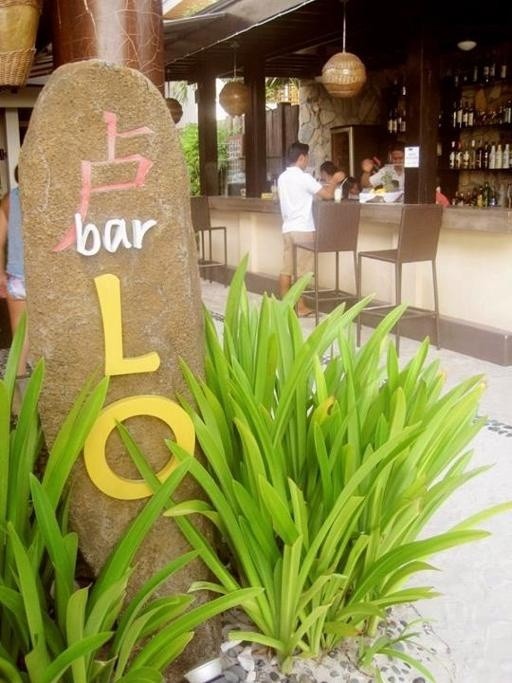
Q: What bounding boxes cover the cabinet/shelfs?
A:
[437,79,512,209]
[330,123,382,178]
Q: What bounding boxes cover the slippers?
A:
[297,310,322,317]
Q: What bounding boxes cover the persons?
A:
[1,185,26,379]
[320,161,357,201]
[361,142,406,194]
[277,140,346,318]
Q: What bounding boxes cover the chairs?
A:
[192,195,228,288]
[355,201,446,357]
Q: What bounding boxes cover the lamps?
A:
[165,72,182,123]
[1,0,43,86]
[457,37,477,52]
[317,2,368,100]
[219,42,253,117]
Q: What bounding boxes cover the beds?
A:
[292,200,365,331]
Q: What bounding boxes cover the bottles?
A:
[451,181,512,208]
[218,167,226,195]
[387,108,406,133]
[449,139,510,169]
[450,99,512,128]
[454,63,507,87]
